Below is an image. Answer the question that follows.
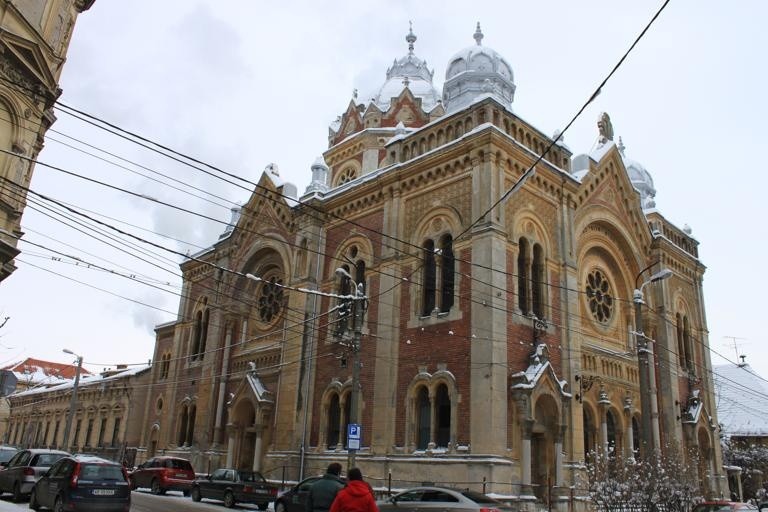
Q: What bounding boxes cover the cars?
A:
[191,467,279,512]
[273,473,378,512]
[375,483,519,512]
[691,500,762,512]
[0,445,21,474]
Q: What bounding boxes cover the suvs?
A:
[26,453,133,512]
[126,455,196,498]
[0,448,80,502]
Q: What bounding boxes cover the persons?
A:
[329,467,380,512]
[730,491,740,502]
[304,463,347,512]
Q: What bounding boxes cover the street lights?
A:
[59,348,85,454]
[630,269,675,490]
[333,267,363,474]
